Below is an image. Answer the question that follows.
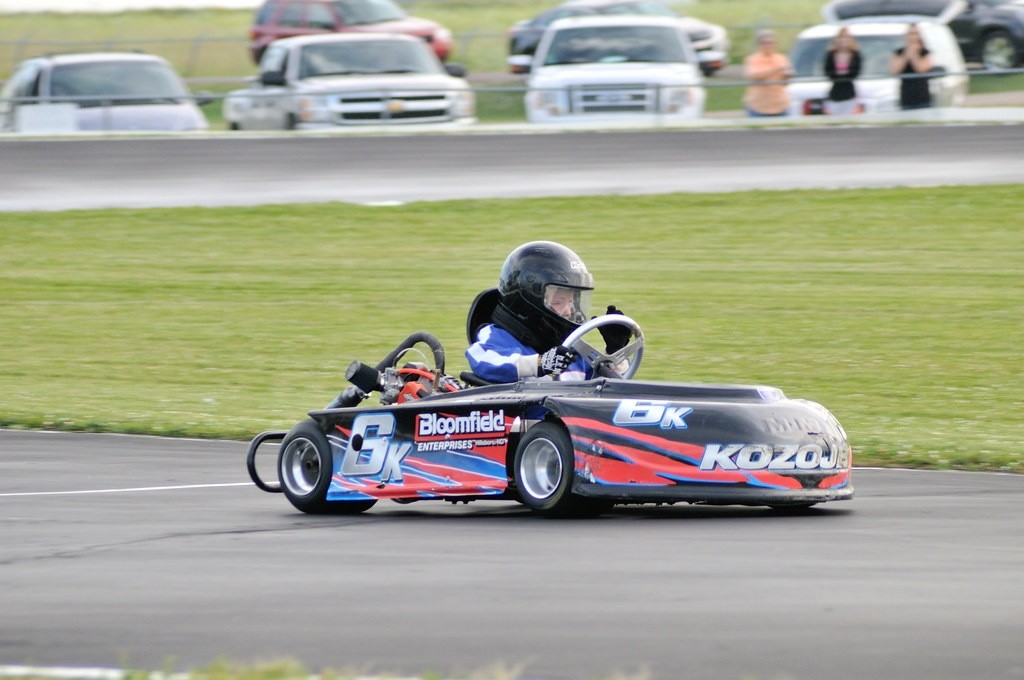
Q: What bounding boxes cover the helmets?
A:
[492,240,595,354]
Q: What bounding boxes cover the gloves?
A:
[538,346,575,376]
[591,305,633,355]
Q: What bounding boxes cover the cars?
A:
[249,0,453,67]
[508,15,705,123]
[506,0,730,74]
[826,0,1024,71]
[223,32,478,130]
[783,21,969,117]
[0,52,215,134]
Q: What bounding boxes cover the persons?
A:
[742,28,793,117]
[889,23,933,108]
[466,241,632,384]
[823,28,862,113]
